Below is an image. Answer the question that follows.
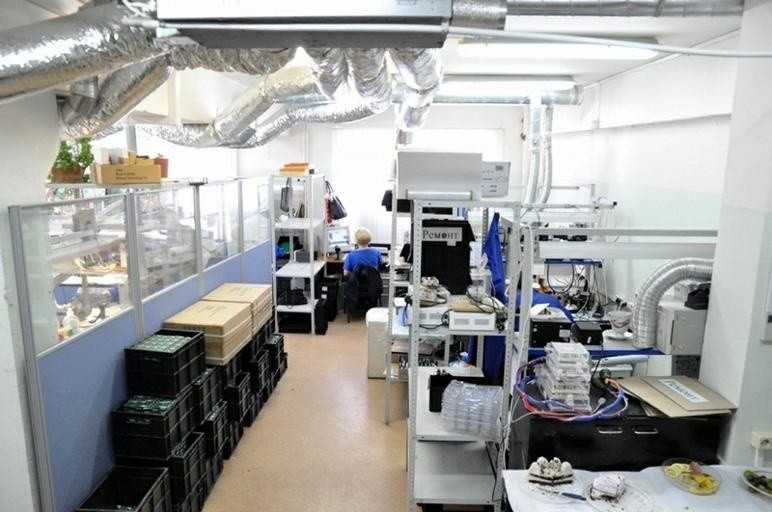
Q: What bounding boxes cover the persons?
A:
[342,229,383,277]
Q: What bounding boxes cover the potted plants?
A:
[47,136,94,184]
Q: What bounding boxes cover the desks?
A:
[326,251,389,279]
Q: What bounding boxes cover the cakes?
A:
[528,456,574,488]
[590,474,627,503]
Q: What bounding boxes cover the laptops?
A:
[327,226,352,253]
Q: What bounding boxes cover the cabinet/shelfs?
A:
[385,283,503,512]
[266,171,327,338]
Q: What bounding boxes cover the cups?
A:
[608,311,634,332]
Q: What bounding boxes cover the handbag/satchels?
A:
[280,187,292,212]
[328,195,346,220]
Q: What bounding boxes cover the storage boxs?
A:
[163,278,275,366]
[90,162,163,185]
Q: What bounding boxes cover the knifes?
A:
[537,485,587,501]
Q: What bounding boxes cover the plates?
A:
[605,330,633,339]
[740,469,772,498]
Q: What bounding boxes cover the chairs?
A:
[342,263,383,323]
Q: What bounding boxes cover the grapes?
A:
[744,469,772,491]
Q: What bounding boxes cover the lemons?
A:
[664,463,690,478]
